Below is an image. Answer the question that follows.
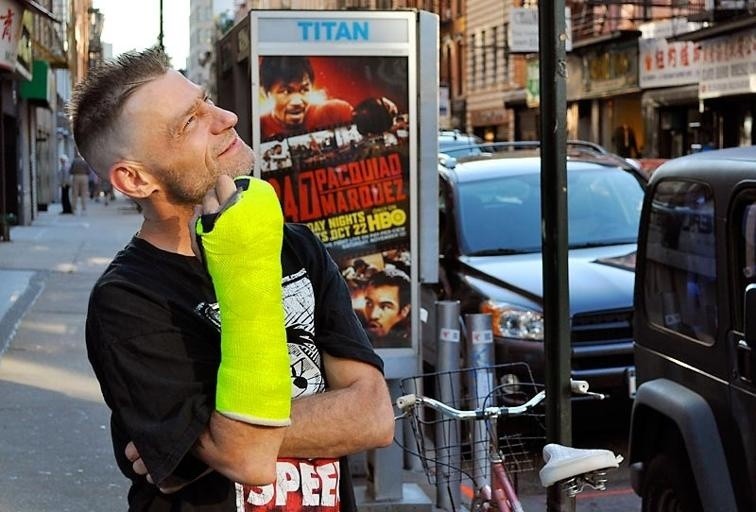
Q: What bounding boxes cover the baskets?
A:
[400,361,546,485]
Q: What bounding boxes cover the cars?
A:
[439,130,492,163]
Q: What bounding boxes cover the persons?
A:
[62,45,396,512]
[259,54,409,170]
[68,154,90,210]
[58,156,73,216]
[612,122,638,161]
[335,247,413,348]
[90,170,116,204]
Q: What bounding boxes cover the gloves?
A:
[352,96,398,137]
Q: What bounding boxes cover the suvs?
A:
[626,145,756,511]
[437,138,661,420]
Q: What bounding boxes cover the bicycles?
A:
[393,361,619,511]
